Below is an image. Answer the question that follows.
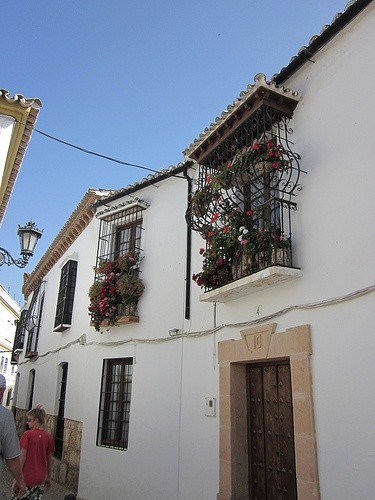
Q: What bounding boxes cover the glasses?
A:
[28,417,36,422]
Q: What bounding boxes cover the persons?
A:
[11,405,55,500]
[0,374,27,500]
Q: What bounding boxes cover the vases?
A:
[242,160,273,180]
[232,246,289,279]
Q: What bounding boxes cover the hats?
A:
[0,374,6,388]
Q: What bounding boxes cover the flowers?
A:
[88,252,144,332]
[187,139,294,290]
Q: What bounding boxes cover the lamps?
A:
[0,219,45,268]
